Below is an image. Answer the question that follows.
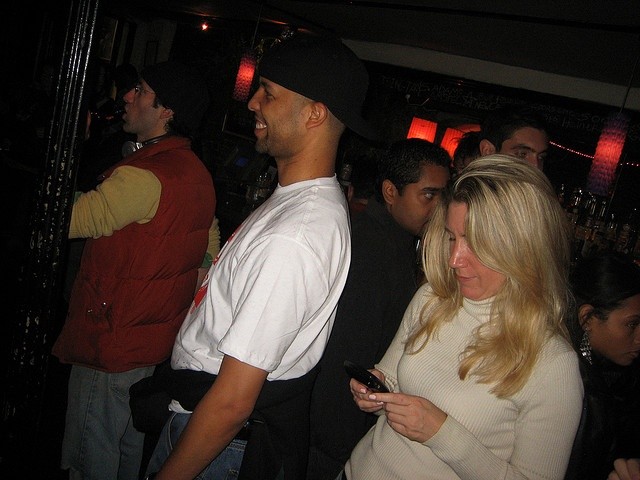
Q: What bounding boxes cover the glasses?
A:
[133,84,157,100]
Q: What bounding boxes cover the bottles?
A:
[90,108,125,133]
[557,181,640,260]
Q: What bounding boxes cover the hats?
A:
[255,30,389,142]
[137,49,208,137]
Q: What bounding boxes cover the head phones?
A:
[121,133,166,159]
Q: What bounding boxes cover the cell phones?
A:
[348,365,389,393]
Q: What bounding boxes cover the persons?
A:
[310,137,451,452]
[453,131,485,172]
[147,36,364,480]
[338,153,584,480]
[479,95,546,177]
[561,254,640,480]
[66,71,221,480]
